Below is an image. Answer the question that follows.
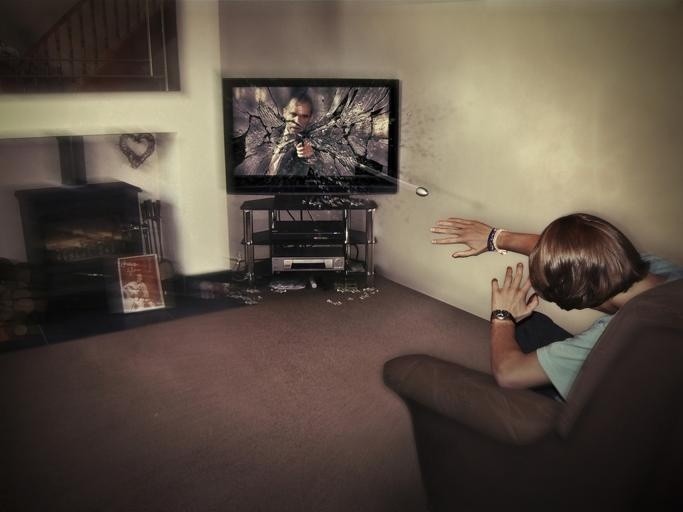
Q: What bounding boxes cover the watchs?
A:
[489,310,516,325]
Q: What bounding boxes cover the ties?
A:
[265,144,284,175]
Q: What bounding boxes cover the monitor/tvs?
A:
[221,78,399,197]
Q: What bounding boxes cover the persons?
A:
[121,270,154,308]
[271,93,317,178]
[429,212,682,404]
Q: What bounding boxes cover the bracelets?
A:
[492,230,508,255]
[486,227,495,252]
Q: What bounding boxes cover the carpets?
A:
[0,277,491,512]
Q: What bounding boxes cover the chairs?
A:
[0,258,50,346]
[384,280,682,511]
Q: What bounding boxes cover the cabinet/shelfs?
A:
[15,181,145,324]
[241,198,380,291]
